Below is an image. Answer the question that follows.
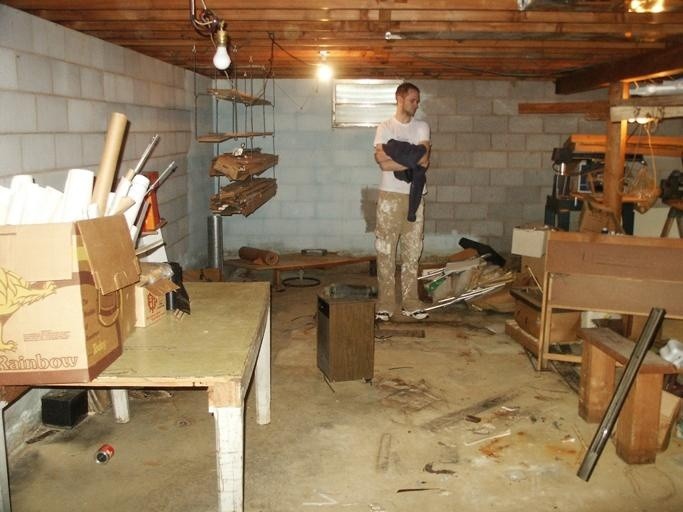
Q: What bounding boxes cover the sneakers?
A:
[375,310,393,322]
[400,309,429,320]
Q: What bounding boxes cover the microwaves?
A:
[567,161,648,197]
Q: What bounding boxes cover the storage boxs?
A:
[135,262,179,329]
[0,216,140,385]
[117,284,139,344]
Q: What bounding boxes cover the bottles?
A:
[160,262,183,311]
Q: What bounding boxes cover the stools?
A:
[575,327,678,464]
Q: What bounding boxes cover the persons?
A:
[371,83,432,324]
[619,151,646,235]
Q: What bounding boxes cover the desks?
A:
[1,281,271,512]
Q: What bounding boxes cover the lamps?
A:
[315,50,334,84]
[629,0,667,14]
[213,19,232,71]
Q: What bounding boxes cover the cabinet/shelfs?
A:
[316,294,378,384]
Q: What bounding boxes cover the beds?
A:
[223,251,377,288]
